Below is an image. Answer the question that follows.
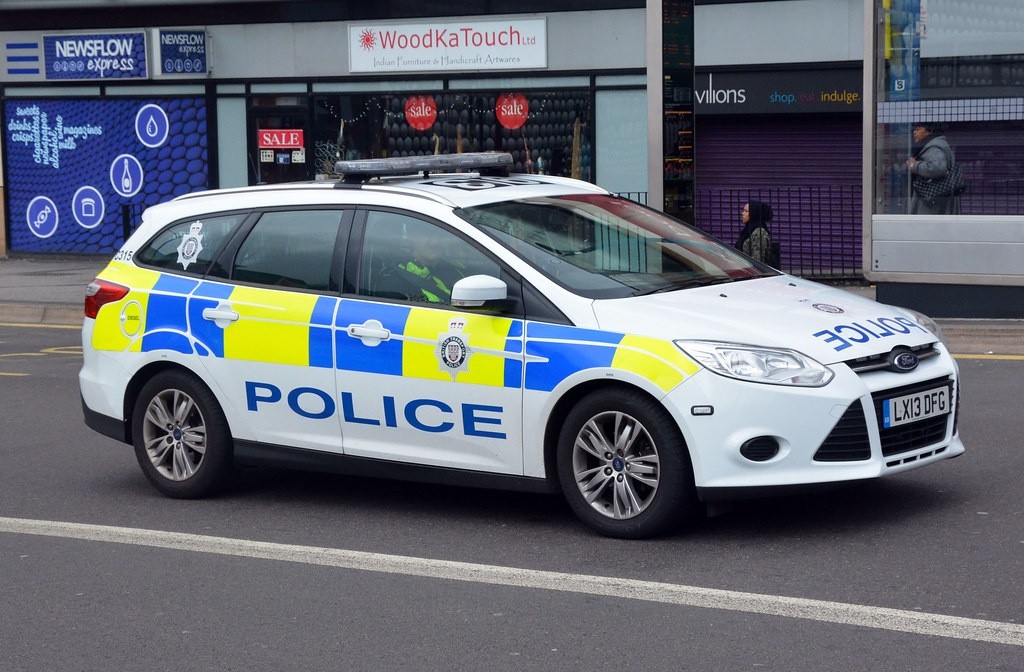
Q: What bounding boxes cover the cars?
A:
[79,151,964,540]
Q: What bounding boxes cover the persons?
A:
[388,220,470,307]
[738,201,773,264]
[906,123,961,215]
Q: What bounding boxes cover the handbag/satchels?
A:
[912,145,967,206]
[768,242,782,272]
